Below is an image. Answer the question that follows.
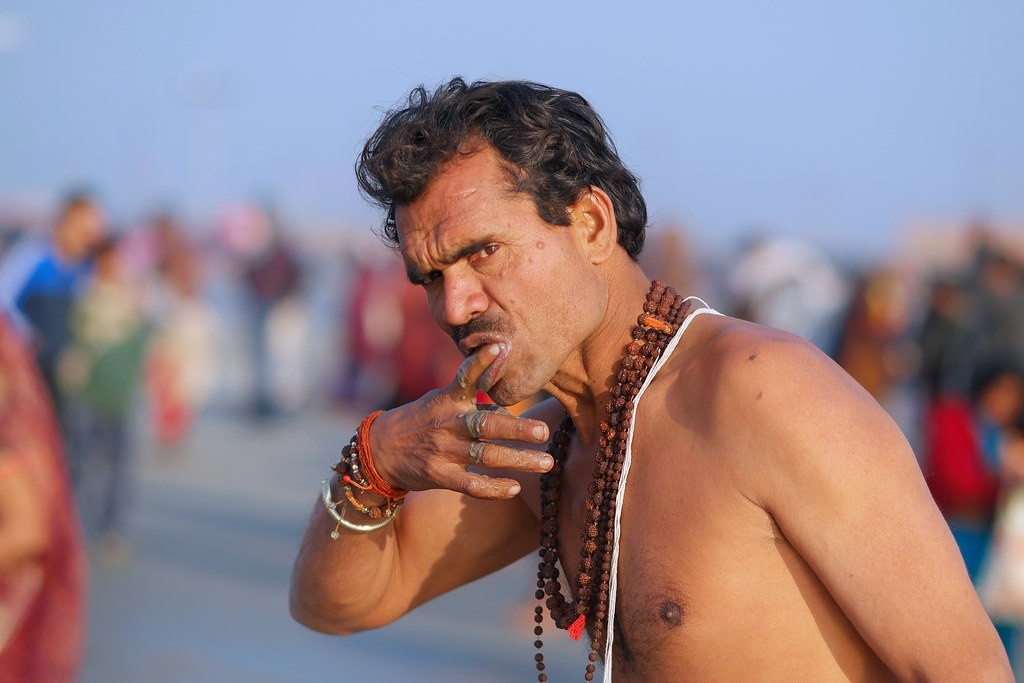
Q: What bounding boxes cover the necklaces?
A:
[535,281,691,682]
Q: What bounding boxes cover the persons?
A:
[723,233,1024,629]
[0,200,303,683]
[288,79,1024,681]
[330,234,465,409]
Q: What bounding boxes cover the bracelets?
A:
[315,410,405,534]
